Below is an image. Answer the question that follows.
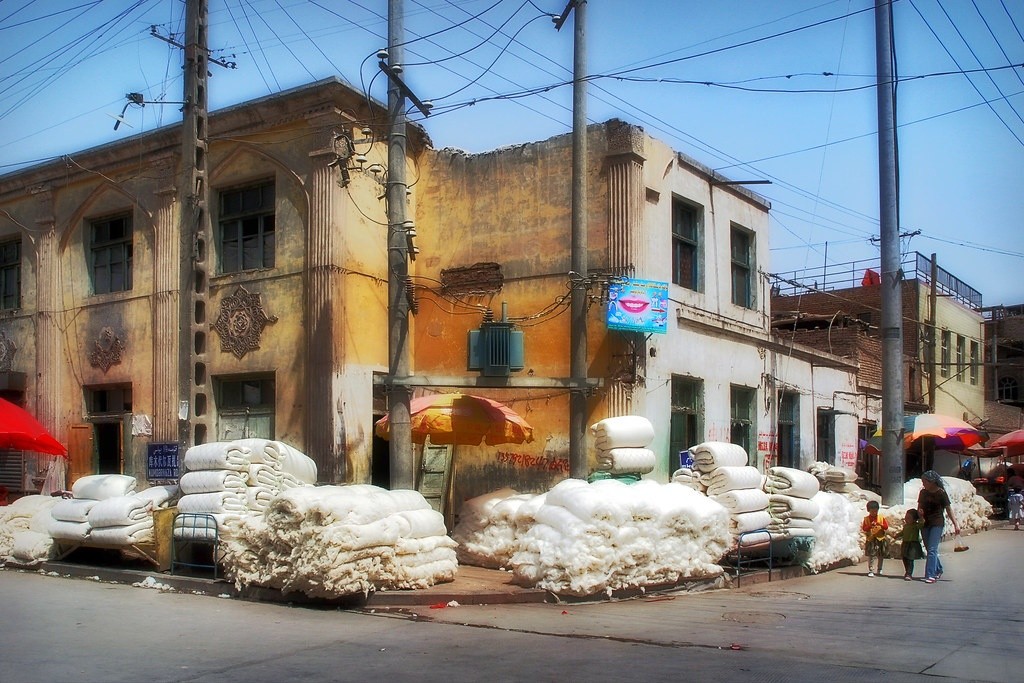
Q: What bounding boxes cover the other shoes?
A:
[1014,522,1020,530]
[905,573,912,581]
[868,572,874,577]
[877,568,882,574]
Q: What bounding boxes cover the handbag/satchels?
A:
[954,533,969,552]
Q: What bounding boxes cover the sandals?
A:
[925,576,936,583]
[936,570,943,578]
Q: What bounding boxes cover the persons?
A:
[860,470,961,583]
[1006,467,1024,530]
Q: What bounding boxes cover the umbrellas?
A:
[863,414,990,473]
[375,393,535,534]
[990,429,1024,448]
[0,395,68,459]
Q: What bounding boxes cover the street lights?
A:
[106,100,215,499]
